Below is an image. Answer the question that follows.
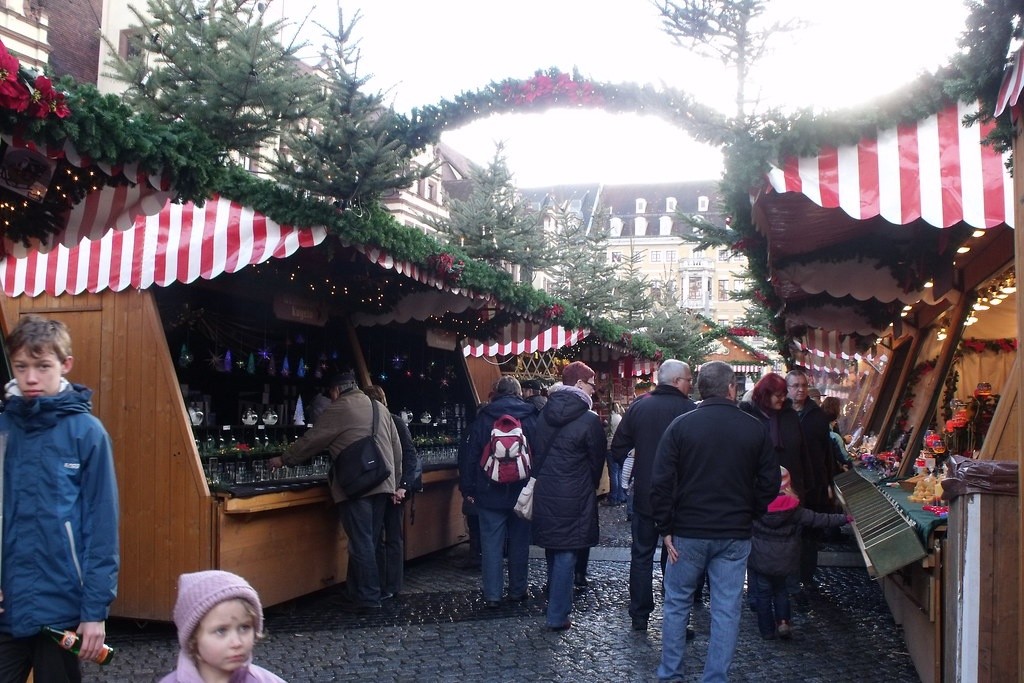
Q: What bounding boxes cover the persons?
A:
[311,380,332,430]
[746,465,854,640]
[601,400,636,506]
[360,386,423,601]
[805,389,851,477]
[984,393,1000,418]
[531,360,608,630]
[649,363,781,683]
[611,358,700,640]
[521,380,548,448]
[457,375,539,608]
[458,400,497,558]
[265,364,402,609]
[0,316,120,683]
[784,369,830,581]
[736,373,815,602]
[158,570,288,683]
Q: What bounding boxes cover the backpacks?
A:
[479,415,533,485]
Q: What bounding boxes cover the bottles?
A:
[41,624,114,665]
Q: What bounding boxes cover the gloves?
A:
[846,515,854,523]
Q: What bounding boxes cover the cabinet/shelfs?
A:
[192,418,466,612]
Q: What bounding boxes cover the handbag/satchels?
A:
[333,435,391,496]
[514,477,537,522]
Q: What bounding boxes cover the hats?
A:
[322,373,353,396]
[780,465,791,489]
[808,389,827,397]
[172,570,262,650]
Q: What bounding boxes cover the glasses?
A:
[681,377,693,384]
[584,380,597,390]
[772,390,787,399]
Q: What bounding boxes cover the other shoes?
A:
[510,592,528,601]
[631,618,648,630]
[487,601,500,608]
[763,633,775,639]
[575,573,588,586]
[686,629,695,639]
[326,591,381,609]
[381,591,395,601]
[777,618,791,638]
[553,621,570,631]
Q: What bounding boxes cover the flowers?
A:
[621,332,632,347]
[729,326,758,337]
[545,304,564,321]
[428,253,464,283]
[755,290,774,307]
[732,236,760,250]
[654,349,661,359]
[503,71,625,117]
[0,38,68,119]
[961,336,1017,353]
[754,352,769,360]
